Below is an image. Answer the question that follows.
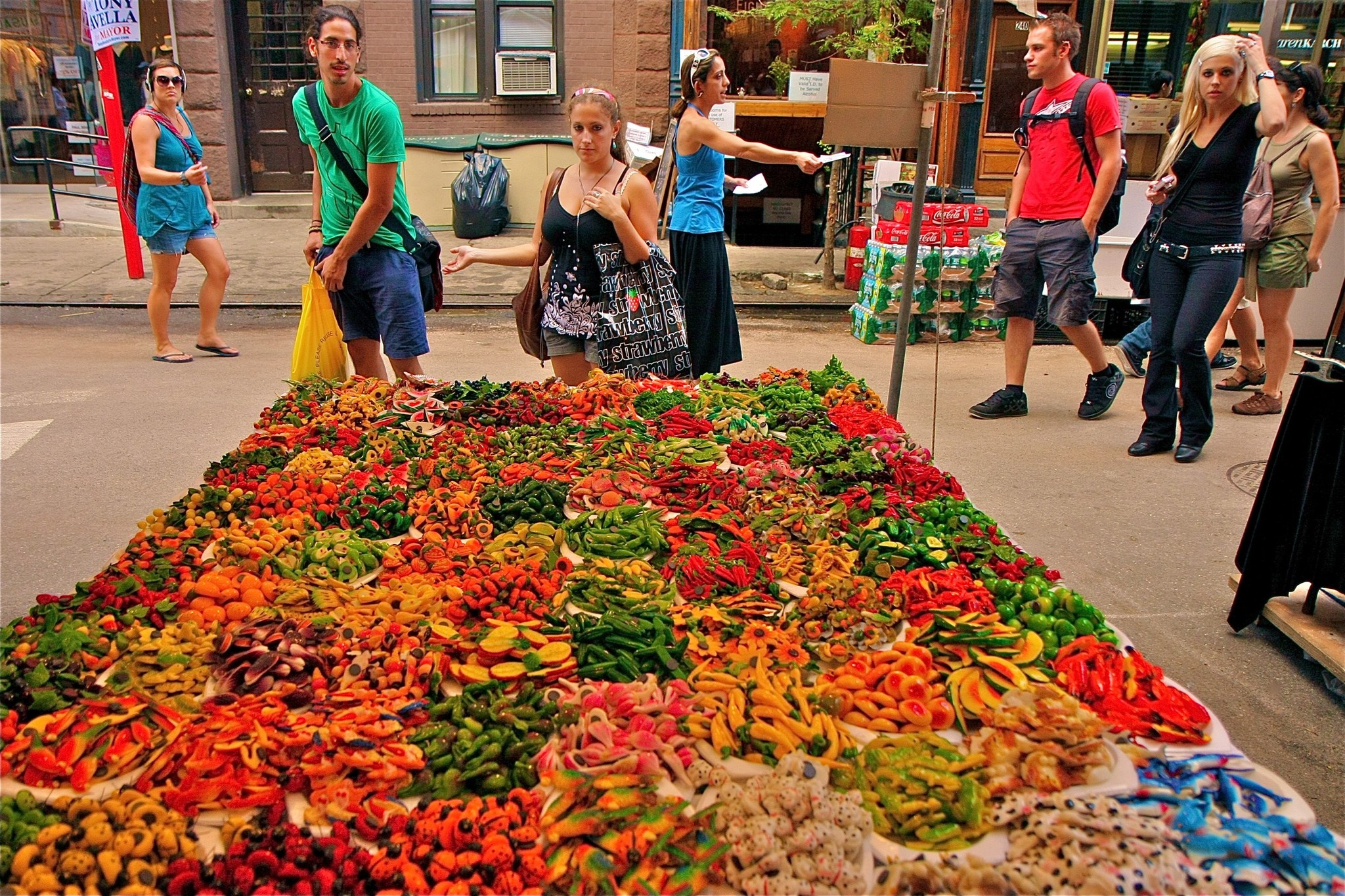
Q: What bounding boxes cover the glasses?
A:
[1287,61,1307,85]
[154,76,184,87]
[316,39,358,53]
[690,48,710,89]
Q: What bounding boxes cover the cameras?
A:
[1148,175,1174,192]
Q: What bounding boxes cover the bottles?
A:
[849,240,1007,332]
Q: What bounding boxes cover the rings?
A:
[593,191,601,198]
[615,192,621,194]
[1249,42,1256,47]
[1152,197,1154,200]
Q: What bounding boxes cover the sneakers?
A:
[1232,390,1282,415]
[1114,344,1146,377]
[967,388,1028,419]
[1077,363,1125,420]
[1212,355,1237,370]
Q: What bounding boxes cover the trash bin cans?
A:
[875,182,964,225]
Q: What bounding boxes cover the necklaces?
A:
[578,159,615,215]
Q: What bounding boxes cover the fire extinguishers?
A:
[843,216,871,291]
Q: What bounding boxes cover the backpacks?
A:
[1242,130,1323,251]
[1021,78,1127,236]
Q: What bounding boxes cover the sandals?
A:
[1216,364,1266,391]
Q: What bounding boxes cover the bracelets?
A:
[312,220,322,225]
[308,225,322,234]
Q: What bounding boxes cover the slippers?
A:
[196,344,237,357]
[152,352,193,363]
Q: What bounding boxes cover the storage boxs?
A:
[1115,94,1174,118]
[1122,134,1161,176]
[1170,99,1183,118]
[1119,115,1169,135]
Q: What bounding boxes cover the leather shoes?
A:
[1175,443,1202,462]
[1128,441,1171,456]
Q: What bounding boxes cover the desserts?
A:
[0,357,1345,896]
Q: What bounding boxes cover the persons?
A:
[112,36,173,127]
[292,4,429,382]
[1113,33,1340,462]
[50,77,93,168]
[669,48,824,385]
[130,59,240,363]
[442,80,658,386]
[969,11,1126,420]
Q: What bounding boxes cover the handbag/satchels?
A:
[1121,211,1164,300]
[408,215,443,313]
[122,111,151,225]
[290,259,350,384]
[510,259,555,361]
[93,126,114,175]
[594,240,693,379]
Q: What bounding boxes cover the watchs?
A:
[1255,70,1275,82]
[180,171,191,187]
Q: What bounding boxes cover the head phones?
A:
[145,58,187,95]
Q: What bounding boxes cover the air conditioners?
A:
[495,49,559,97]
